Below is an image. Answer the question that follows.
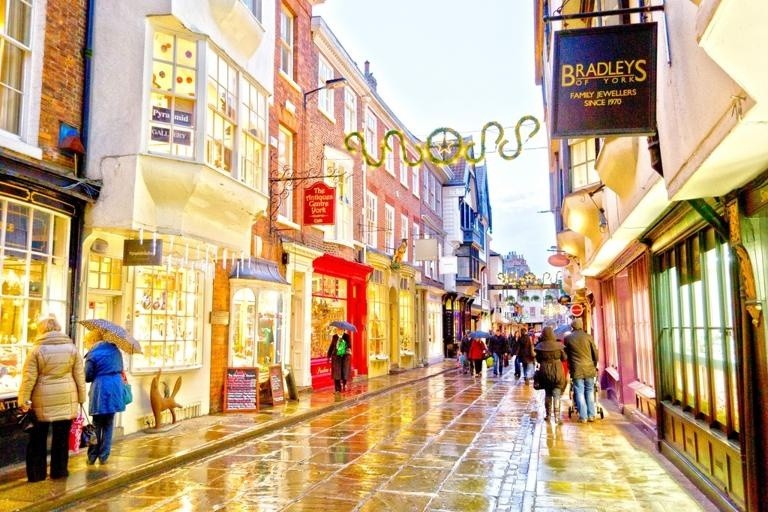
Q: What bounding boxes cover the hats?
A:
[571,318,583,330]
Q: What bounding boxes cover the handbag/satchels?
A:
[485,352,495,368]
[123,382,134,405]
[533,363,548,391]
[79,403,99,450]
[68,404,86,455]
[456,352,465,369]
[503,353,509,367]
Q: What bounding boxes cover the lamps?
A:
[590,132,642,203]
[554,230,587,265]
[559,187,603,246]
[300,77,346,111]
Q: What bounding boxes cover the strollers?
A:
[565,372,604,421]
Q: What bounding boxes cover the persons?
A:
[83,330,126,466]
[459,318,573,385]
[16,313,88,483]
[564,318,599,422]
[327,328,352,394]
[534,327,568,424]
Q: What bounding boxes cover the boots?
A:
[553,398,565,425]
[543,396,553,421]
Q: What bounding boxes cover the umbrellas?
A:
[330,321,357,334]
[78,316,143,355]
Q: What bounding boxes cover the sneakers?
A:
[576,415,596,424]
[459,368,530,387]
[85,459,96,467]
[97,459,108,466]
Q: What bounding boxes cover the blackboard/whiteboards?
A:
[223,366,259,413]
[269,364,286,406]
